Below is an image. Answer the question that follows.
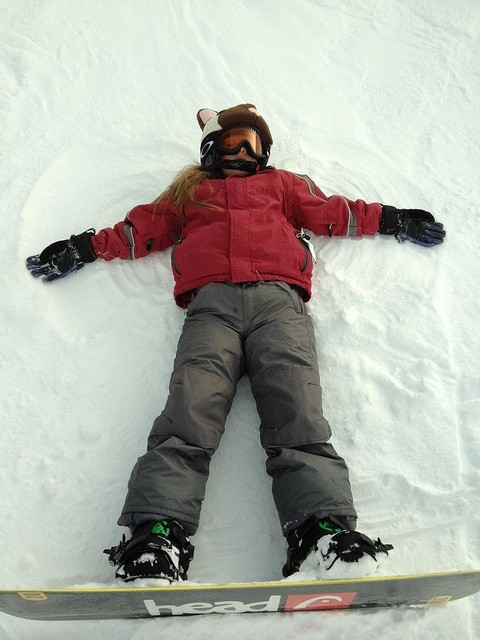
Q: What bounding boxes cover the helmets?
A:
[200,103,273,175]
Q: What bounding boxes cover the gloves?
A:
[26,228,96,283]
[380,204,445,247]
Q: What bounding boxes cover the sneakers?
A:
[104,519,194,583]
[280,516,395,577]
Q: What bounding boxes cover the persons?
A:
[27,103,446,582]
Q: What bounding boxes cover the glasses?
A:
[201,127,270,164]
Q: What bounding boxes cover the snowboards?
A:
[1,570,479,620]
